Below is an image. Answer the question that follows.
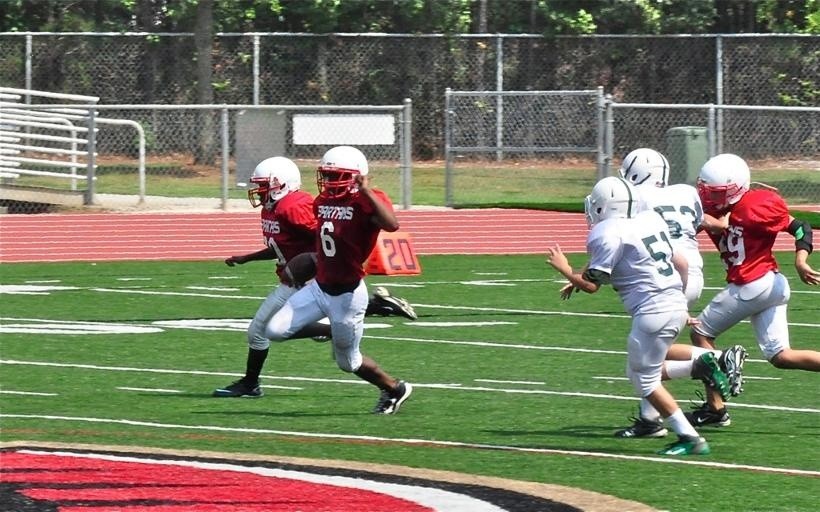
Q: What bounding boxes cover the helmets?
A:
[616,147,671,191]
[246,154,303,211]
[315,144,370,203]
[696,152,751,214]
[582,175,645,230]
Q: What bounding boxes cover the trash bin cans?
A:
[666,127,709,189]
[238,109,287,188]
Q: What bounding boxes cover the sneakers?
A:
[655,433,716,458]
[690,350,733,402]
[212,373,266,400]
[612,412,669,441]
[716,344,750,398]
[372,376,417,416]
[370,285,421,322]
[683,403,735,430]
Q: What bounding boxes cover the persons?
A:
[211,156,417,398]
[546,147,749,457]
[264,146,413,415]
[683,153,820,426]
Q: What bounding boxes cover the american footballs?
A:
[282,252,319,283]
